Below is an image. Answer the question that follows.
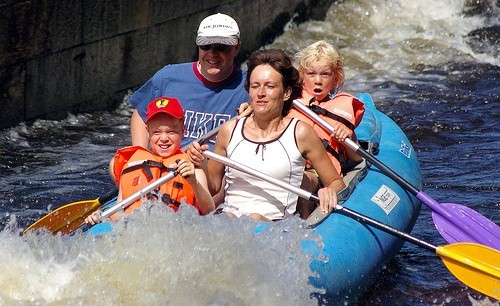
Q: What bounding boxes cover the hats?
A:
[196,13,240,45]
[146,96,185,121]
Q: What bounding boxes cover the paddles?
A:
[193,138,500,304]
[66,158,190,237]
[292,97,500,247]
[23,108,248,237]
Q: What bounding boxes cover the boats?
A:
[80,90,423,306]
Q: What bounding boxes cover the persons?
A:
[186,49,346,223]
[286,40,364,219]
[128,13,253,154]
[84,97,216,226]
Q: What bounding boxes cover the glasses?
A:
[199,43,235,52]
